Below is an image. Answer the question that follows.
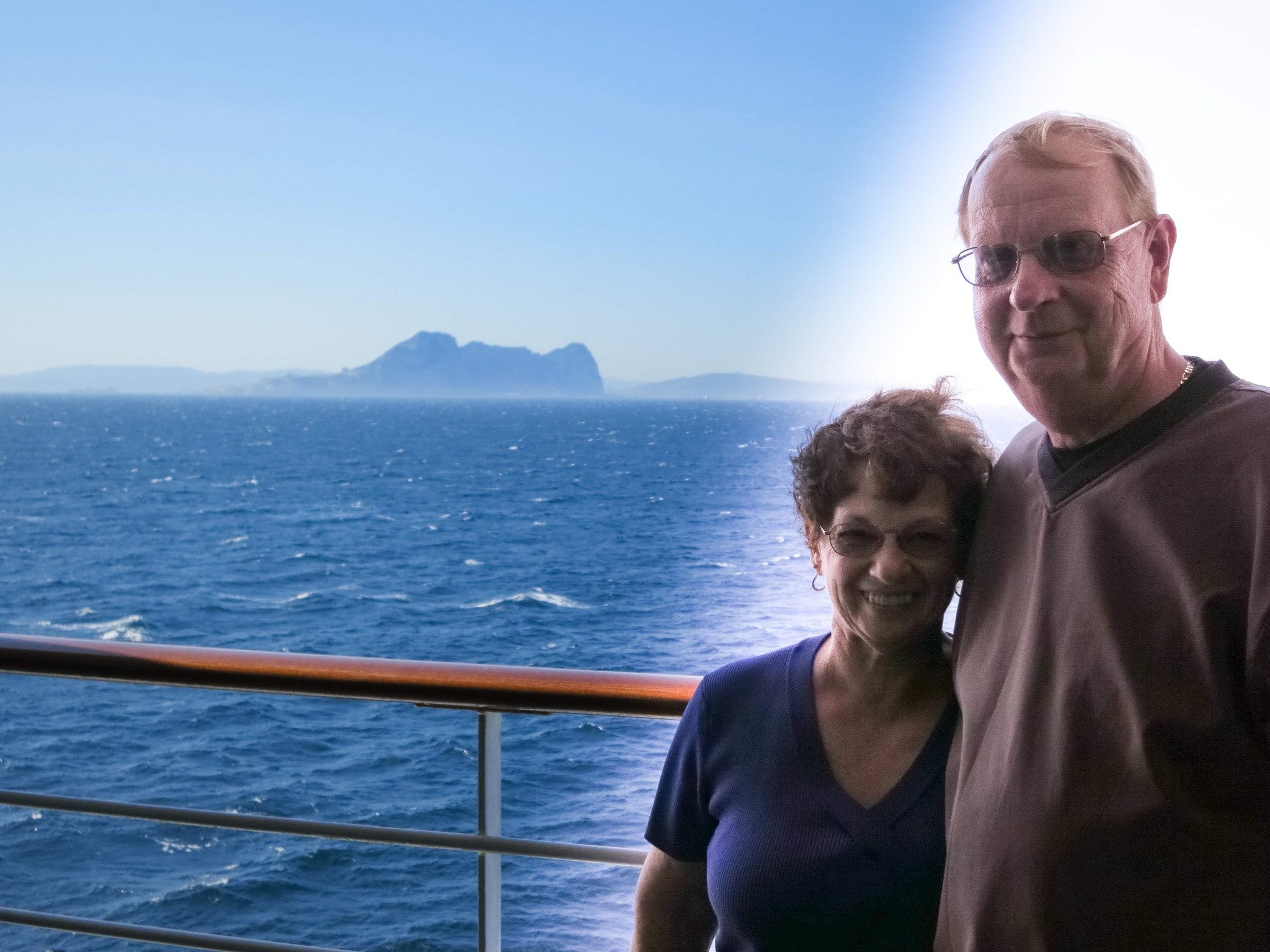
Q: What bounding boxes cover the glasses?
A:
[819,522,960,558]
[951,218,1141,287]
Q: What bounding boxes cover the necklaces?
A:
[1178,360,1194,387]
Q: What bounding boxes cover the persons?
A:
[930,113,1270,952]
[630,390,998,952]
